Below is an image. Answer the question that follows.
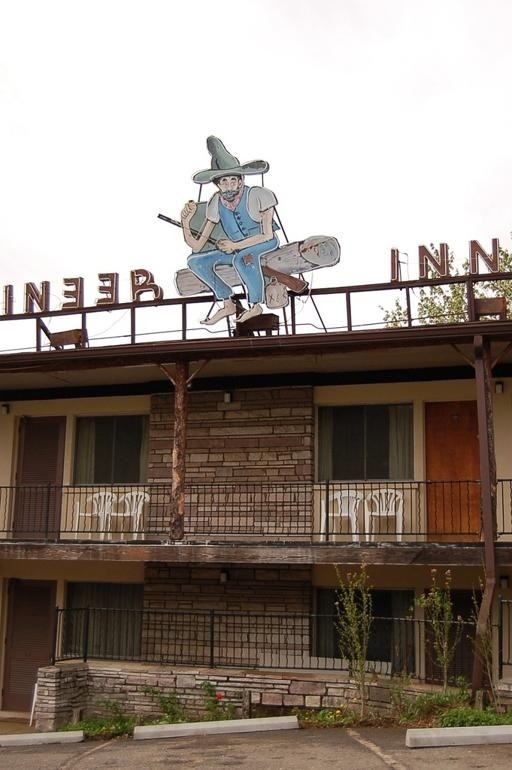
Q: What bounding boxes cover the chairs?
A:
[321,489,405,543]
[74,491,150,541]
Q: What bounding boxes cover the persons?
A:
[181,135,279,325]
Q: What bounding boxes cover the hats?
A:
[192,134,270,184]
